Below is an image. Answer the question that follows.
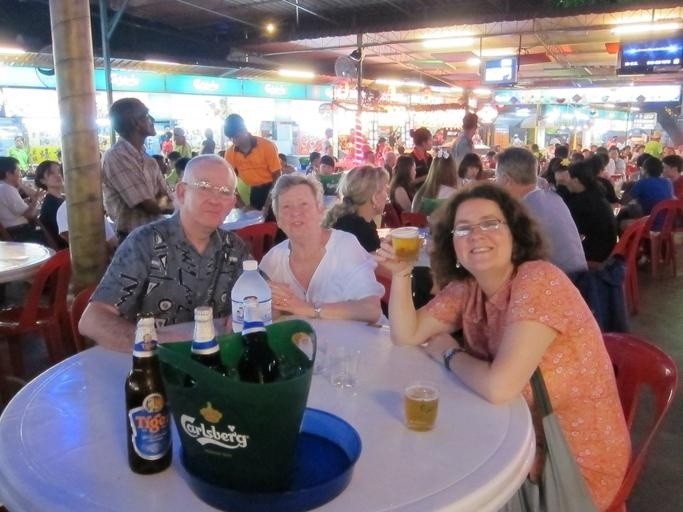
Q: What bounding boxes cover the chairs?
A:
[0,246,69,369]
[600,330,677,511]
[69,284,96,352]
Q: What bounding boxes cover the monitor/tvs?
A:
[615,37,682,77]
[480,55,518,87]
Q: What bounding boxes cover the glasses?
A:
[133,109,150,126]
[449,216,508,238]
[183,179,237,197]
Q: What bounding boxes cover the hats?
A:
[464,112,478,122]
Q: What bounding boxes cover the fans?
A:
[334,57,358,84]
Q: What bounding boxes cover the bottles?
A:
[230,260,272,331]
[183,305,228,387]
[121,309,173,473]
[237,295,280,385]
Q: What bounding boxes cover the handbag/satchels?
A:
[504,367,599,511]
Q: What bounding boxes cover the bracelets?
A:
[392,273,413,279]
[443,346,466,373]
[314,301,321,320]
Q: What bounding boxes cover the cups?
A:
[389,224,420,264]
[403,380,440,434]
[311,338,362,389]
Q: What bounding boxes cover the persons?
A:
[482,131,683,292]
[75,153,272,357]
[259,172,386,325]
[97,98,175,240]
[56,196,120,252]
[371,181,633,511]
[0,136,69,249]
[299,112,480,254]
[150,114,296,246]
